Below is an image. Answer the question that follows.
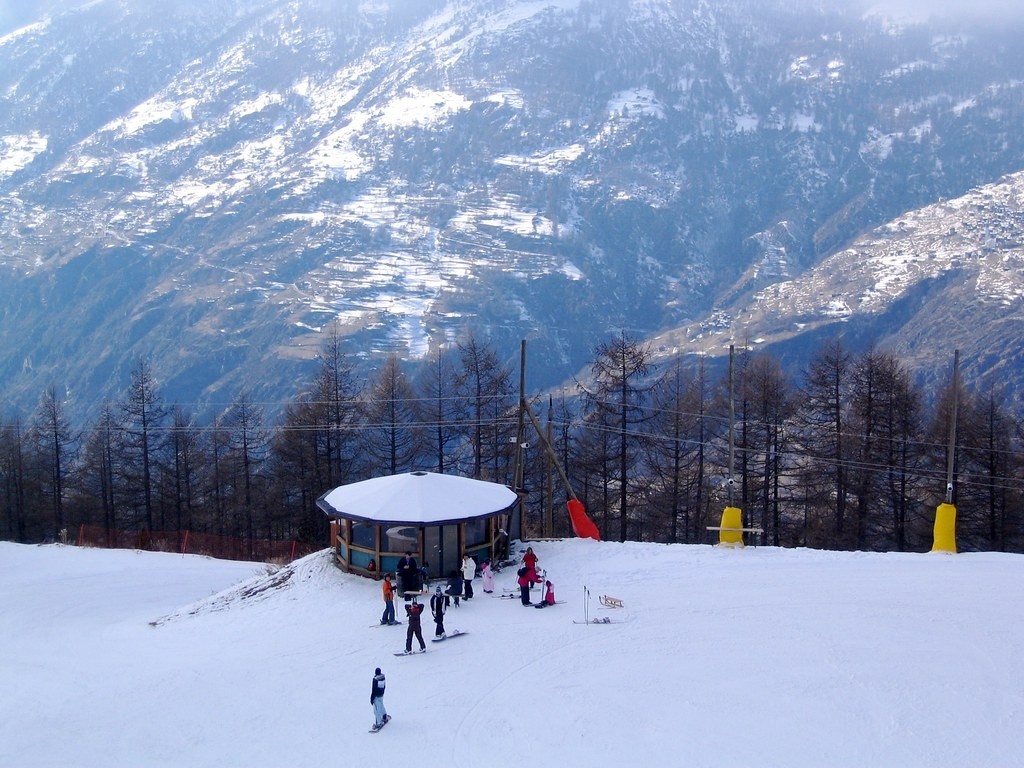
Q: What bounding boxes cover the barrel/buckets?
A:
[418,573,424,591]
[395,571,403,597]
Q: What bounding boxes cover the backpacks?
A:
[517,567,531,577]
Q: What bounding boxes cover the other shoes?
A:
[419,648,426,652]
[372,724,380,730]
[468,595,472,598]
[381,620,388,624]
[382,714,387,721]
[389,620,398,624]
[462,596,468,601]
[522,601,532,606]
[436,632,446,639]
[404,650,412,654]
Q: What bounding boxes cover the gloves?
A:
[390,586,397,591]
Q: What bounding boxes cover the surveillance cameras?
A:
[521,443,529,448]
[510,437,517,443]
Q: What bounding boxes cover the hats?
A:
[436,586,441,592]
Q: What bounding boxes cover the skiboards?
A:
[369,618,409,629]
[503,587,533,592]
[493,593,521,599]
[572,617,623,625]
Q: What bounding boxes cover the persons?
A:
[459,553,476,601]
[370,668,387,728]
[404,602,426,653]
[481,558,494,593]
[382,572,398,623]
[518,566,544,605]
[430,586,446,637]
[546,580,554,606]
[397,551,417,601]
[521,547,538,588]
[445,570,462,605]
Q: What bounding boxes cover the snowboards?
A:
[431,629,468,643]
[390,649,429,656]
[368,714,391,732]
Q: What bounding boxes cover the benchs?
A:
[706,526,765,548]
[405,591,466,608]
